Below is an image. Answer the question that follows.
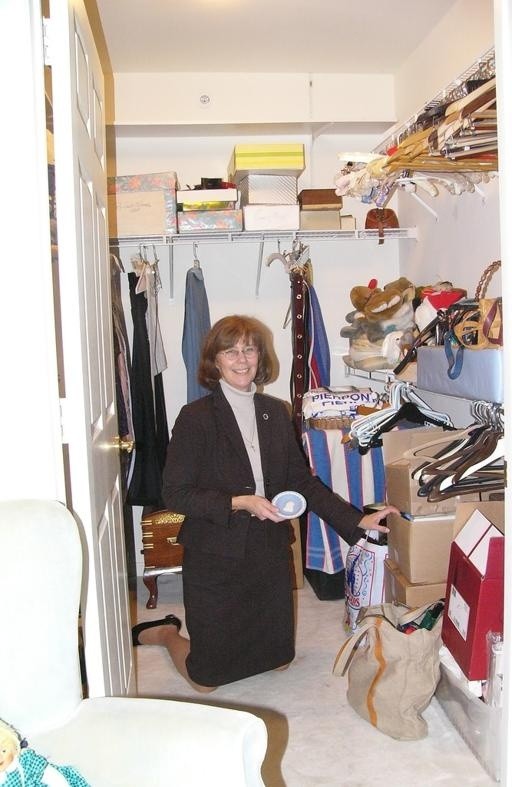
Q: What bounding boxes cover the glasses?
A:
[217,347,260,358]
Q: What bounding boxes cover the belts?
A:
[294,275,304,430]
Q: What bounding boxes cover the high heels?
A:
[133,614,182,645]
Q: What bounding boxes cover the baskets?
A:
[309,418,353,431]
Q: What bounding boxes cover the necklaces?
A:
[240,415,258,454]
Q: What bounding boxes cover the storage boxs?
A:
[103,145,305,238]
[382,426,502,683]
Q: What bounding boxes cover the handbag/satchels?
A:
[365,208,400,244]
[333,600,445,742]
[435,262,502,350]
[344,523,389,635]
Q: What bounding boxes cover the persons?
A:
[0,717,92,787]
[130,314,403,695]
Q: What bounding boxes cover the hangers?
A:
[334,56,497,211]
[343,378,503,503]
